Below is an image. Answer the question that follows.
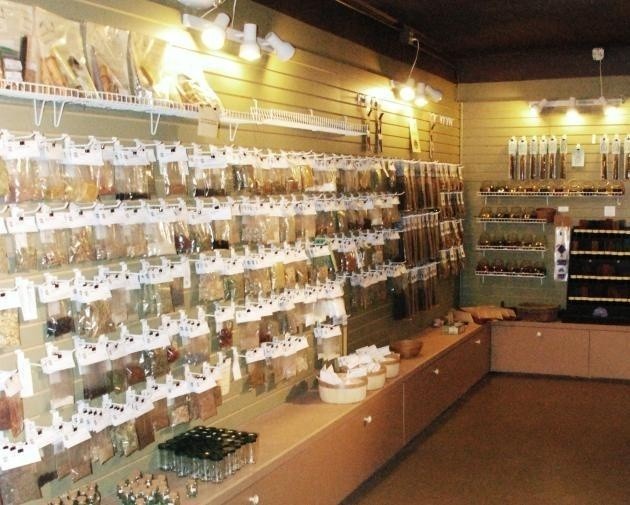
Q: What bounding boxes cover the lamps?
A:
[425,85,443,103]
[399,77,417,101]
[415,82,427,106]
[183,12,296,62]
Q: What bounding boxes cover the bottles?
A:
[159,425,258,483]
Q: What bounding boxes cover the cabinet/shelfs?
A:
[462,317,490,395]
[473,99,628,323]
[489,318,629,380]
[51,365,403,505]
[394,306,466,447]
[1,77,466,505]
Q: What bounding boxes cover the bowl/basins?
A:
[536,208,556,222]
[318,339,424,406]
